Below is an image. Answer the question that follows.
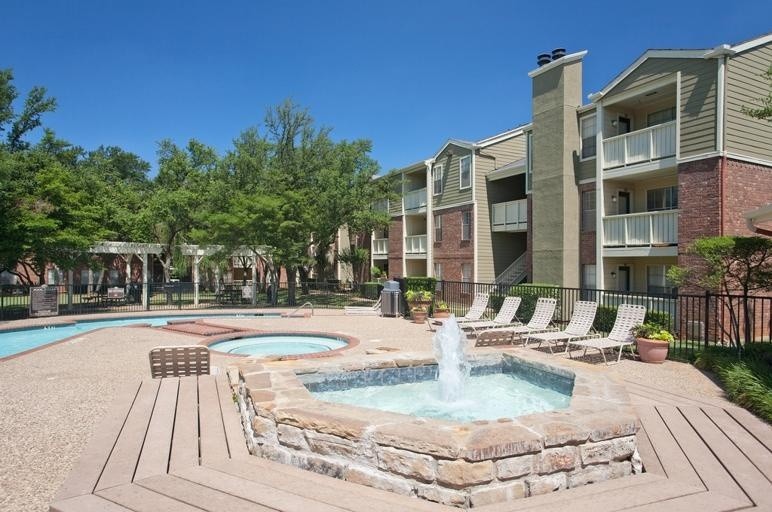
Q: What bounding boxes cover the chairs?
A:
[344,295,381,317]
[424,291,648,366]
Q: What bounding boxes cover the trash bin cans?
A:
[381,289,402,318]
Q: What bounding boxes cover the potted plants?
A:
[629,322,676,362]
[402,289,450,325]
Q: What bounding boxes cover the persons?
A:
[132,278,141,303]
